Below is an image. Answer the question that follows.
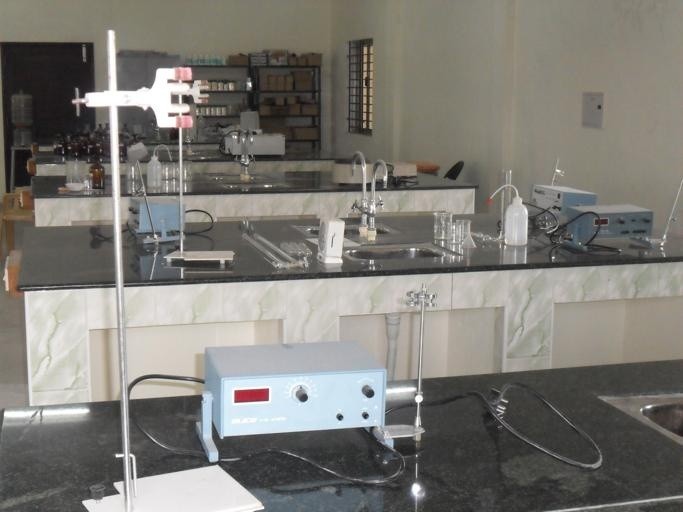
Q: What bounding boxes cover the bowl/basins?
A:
[65,183,83,192]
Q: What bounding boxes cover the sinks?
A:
[599,389,683,445]
[228,183,289,192]
[304,219,387,237]
[213,173,274,183]
[344,243,449,268]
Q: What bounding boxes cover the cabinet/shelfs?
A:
[183,65,320,143]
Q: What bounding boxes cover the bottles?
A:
[488,184,530,246]
[245,78,252,90]
[207,79,236,91]
[54,119,130,163]
[496,168,513,243]
[197,105,227,116]
[89,156,105,188]
[185,54,226,66]
[146,144,173,187]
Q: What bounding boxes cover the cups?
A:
[432,211,472,242]
[160,160,192,184]
[66,161,86,184]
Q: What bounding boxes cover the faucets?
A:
[241,129,255,176]
[236,128,244,164]
[351,150,367,230]
[368,159,388,239]
